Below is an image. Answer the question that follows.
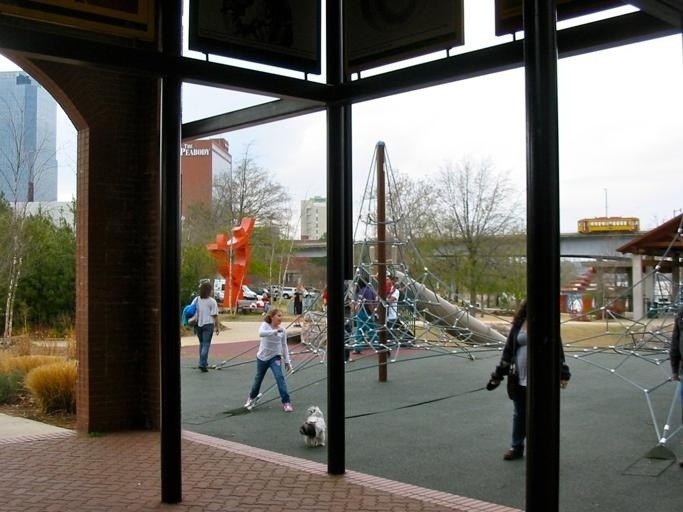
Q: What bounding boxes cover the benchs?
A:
[236,300,265,314]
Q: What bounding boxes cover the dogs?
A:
[300,405,326,448]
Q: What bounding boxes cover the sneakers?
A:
[282,402,292,412]
[199,366,207,373]
[245,398,255,410]
[504,447,524,461]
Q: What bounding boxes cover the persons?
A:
[668,304,683,471]
[289,279,305,326]
[322,284,354,363]
[244,309,293,413]
[347,274,381,353]
[374,272,401,358]
[190,282,221,373]
[485,296,571,461]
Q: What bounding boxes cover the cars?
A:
[198,278,320,313]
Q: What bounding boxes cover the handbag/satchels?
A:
[184,305,197,326]
